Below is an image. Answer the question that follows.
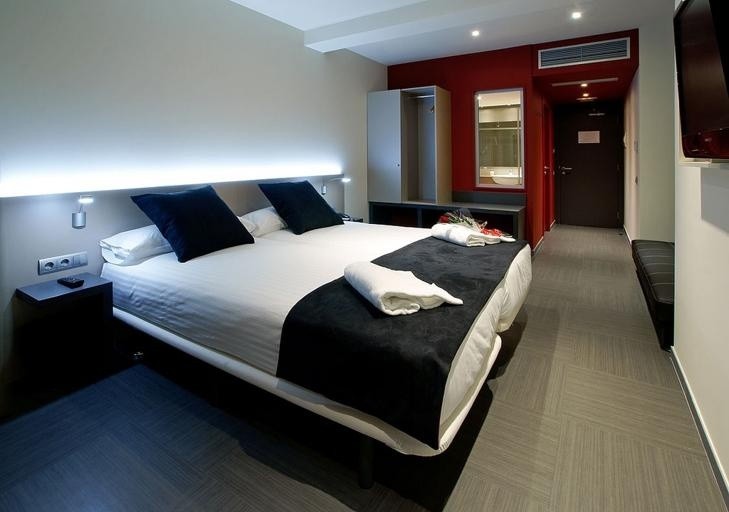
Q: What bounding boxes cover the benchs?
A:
[630,240,675,350]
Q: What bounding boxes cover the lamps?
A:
[322,177,352,194]
[72,195,94,228]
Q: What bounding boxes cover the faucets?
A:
[508,169,512,175]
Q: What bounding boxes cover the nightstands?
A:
[343,218,364,222]
[16,273,113,405]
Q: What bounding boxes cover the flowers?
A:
[440,208,512,238]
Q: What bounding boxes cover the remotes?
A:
[59,276,84,288]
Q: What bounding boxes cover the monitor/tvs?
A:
[673,0,729,159]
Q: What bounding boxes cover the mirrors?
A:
[474,87,525,189]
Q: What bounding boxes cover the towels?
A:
[344,260,464,315]
[432,224,516,247]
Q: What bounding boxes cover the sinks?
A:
[491,175,522,185]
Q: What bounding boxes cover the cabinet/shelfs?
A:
[367,85,452,206]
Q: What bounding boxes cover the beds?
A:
[100,220,533,478]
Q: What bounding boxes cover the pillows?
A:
[99,216,256,266]
[130,184,254,262]
[257,180,345,234]
[242,206,289,237]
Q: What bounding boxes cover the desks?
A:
[369,201,527,239]
